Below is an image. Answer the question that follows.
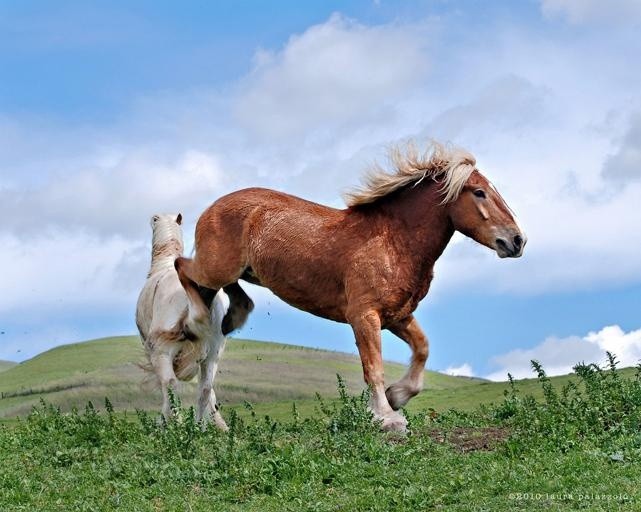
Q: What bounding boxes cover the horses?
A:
[133,211,230,437]
[172,134,529,440]
